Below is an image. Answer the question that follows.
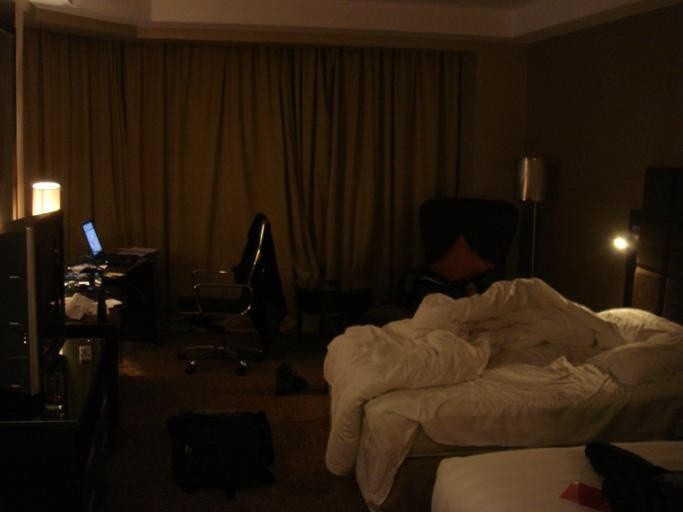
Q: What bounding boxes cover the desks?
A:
[64,246,174,347]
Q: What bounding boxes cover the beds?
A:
[432,441,681,512]
[325,308,680,509]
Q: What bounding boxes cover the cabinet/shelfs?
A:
[0,335,121,512]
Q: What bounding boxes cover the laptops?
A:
[81,220,139,266]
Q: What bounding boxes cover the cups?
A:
[42,372,65,409]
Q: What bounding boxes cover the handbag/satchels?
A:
[164,410,277,494]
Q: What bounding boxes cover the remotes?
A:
[79,345,93,364]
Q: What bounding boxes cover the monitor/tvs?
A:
[1,208,66,399]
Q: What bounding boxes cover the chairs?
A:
[175,214,276,378]
[404,195,521,313]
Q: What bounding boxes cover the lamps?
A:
[30,180,61,217]
[514,155,545,279]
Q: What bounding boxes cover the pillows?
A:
[427,233,495,286]
